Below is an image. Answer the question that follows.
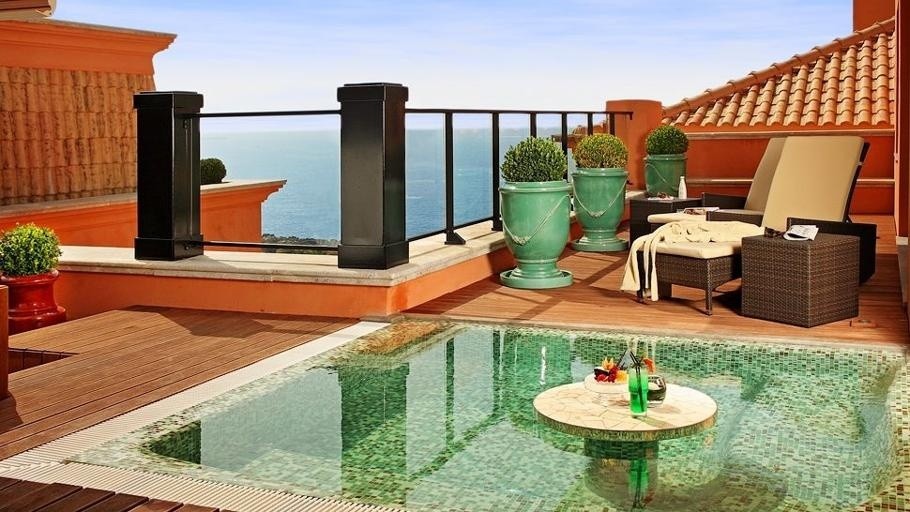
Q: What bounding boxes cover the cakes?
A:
[584,356,630,394]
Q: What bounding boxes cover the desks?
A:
[739,229,863,328]
[629,195,702,254]
[532,378,719,500]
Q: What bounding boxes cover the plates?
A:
[585,374,628,393]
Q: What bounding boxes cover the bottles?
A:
[678,176,687,200]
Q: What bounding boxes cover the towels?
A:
[619,219,768,303]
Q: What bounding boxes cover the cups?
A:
[627,365,648,415]
[647,373,666,407]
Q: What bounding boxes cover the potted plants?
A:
[642,125,689,197]
[0,220,67,334]
[571,131,629,252]
[496,135,574,291]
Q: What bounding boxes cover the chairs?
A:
[630,137,880,316]
